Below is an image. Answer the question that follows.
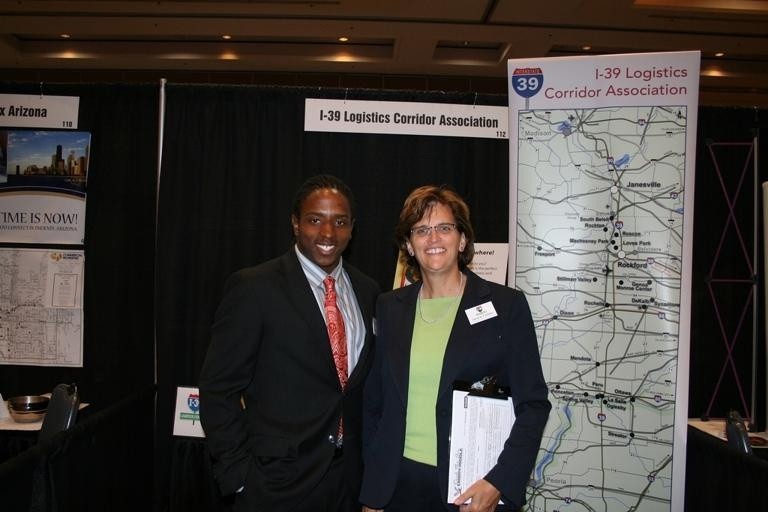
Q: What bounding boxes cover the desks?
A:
[688,413,768,512]
[0,377,159,512]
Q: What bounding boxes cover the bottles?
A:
[727,410,752,457]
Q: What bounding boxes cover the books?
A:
[447,383,516,505]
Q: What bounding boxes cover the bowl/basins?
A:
[7,395,50,424]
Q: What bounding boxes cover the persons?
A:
[358,184,552,512]
[196,173,379,511]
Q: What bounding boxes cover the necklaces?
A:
[418,271,463,325]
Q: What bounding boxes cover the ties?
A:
[323,275,349,392]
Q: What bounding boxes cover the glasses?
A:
[410,223,457,236]
